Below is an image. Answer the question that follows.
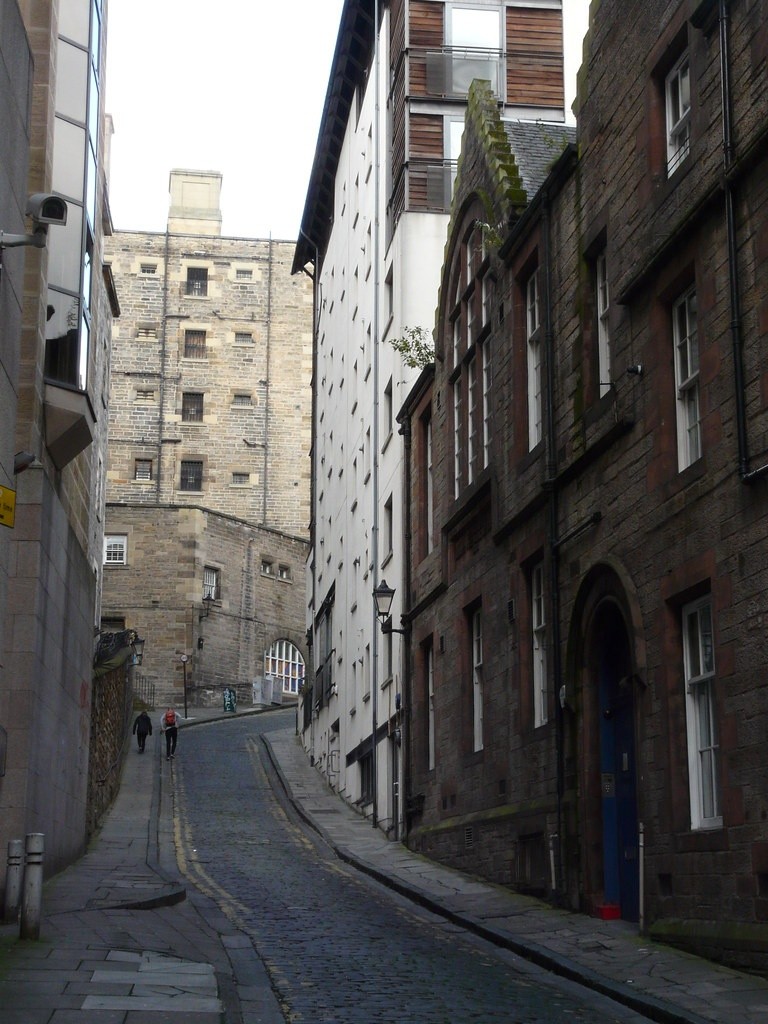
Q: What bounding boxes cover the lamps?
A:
[373,580,404,634]
[199,591,215,622]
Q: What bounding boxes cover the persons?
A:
[132,710,152,754]
[160,707,181,761]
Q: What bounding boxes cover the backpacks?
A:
[166,711,175,726]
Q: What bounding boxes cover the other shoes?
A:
[171,754,175,758]
[166,757,170,760]
[139,747,142,751]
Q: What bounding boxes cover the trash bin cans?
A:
[222,686,237,713]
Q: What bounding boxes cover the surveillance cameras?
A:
[24,194,67,226]
